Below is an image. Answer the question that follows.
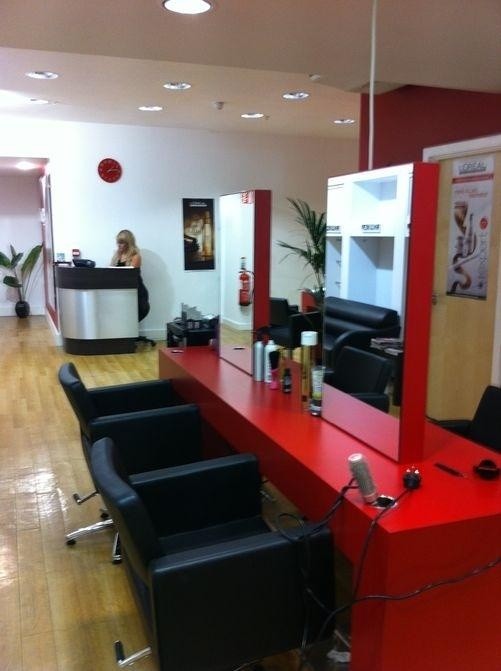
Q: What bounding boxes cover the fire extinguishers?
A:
[238,267,256,306]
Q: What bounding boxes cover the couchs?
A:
[324,295,402,369]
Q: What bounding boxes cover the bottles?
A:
[299,331,318,410]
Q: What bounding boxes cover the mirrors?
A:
[322,160,441,465]
[218,190,272,377]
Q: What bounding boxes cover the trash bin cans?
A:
[300,635,351,671]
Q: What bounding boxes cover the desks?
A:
[364,345,403,407]
[166,321,217,348]
[158,348,501,671]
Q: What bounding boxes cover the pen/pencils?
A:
[436,463,459,476]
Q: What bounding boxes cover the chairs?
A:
[59,362,236,565]
[431,386,501,453]
[269,297,323,351]
[60,437,353,670]
[324,344,392,414]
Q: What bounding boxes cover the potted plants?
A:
[1,243,45,318]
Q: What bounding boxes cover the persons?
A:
[109,228,149,302]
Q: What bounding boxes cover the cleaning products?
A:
[253,336,275,385]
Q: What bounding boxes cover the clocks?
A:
[98,159,122,184]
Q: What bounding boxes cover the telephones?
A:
[72,259,95,268]
